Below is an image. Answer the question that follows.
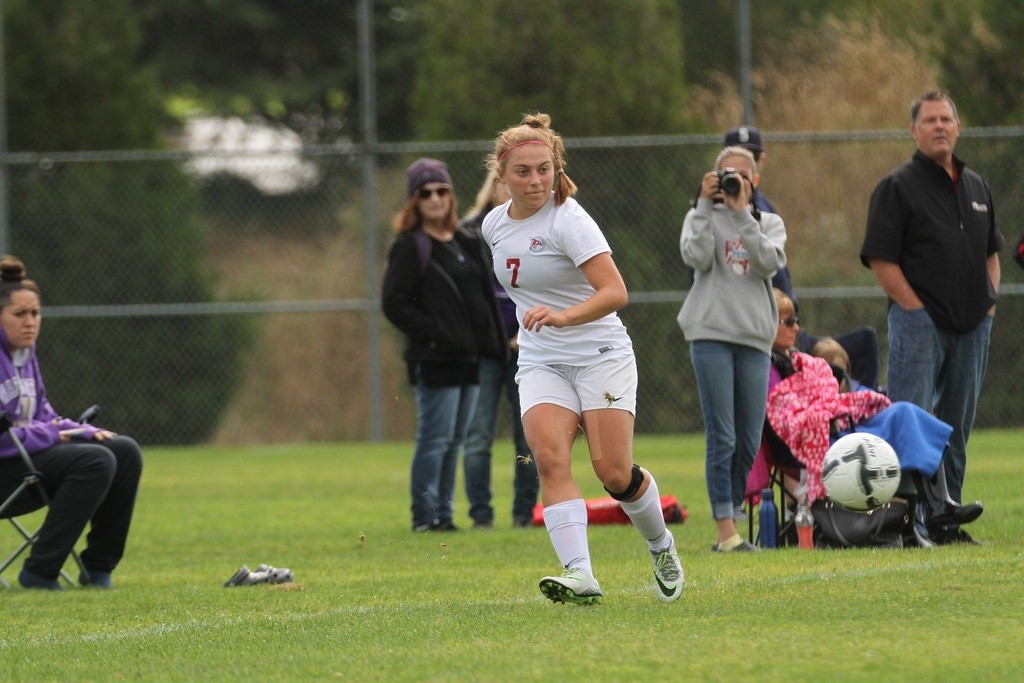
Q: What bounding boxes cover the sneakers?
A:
[649,529,684,604]
[539,567,603,605]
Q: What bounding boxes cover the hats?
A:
[408,157,453,196]
[725,126,762,152]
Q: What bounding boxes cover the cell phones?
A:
[60,428,85,436]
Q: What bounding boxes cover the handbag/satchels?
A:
[811,496,909,549]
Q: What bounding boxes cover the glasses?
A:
[419,187,449,199]
[752,150,760,161]
[777,318,800,327]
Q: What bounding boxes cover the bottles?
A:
[758,488,780,548]
[795,501,814,550]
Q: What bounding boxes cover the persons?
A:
[380,158,540,534]
[481,111,685,606]
[723,124,983,548]
[0,256,143,592]
[860,88,1004,546]
[676,143,787,555]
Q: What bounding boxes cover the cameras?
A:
[716,167,741,195]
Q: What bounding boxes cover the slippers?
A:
[711,538,763,553]
[256,564,294,584]
[224,563,276,588]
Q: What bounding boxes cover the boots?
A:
[897,492,932,548]
[919,457,983,525]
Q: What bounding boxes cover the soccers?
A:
[821,431,902,514]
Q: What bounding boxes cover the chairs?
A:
[0,406,100,589]
[748,326,881,547]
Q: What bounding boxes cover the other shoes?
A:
[78,568,113,589]
[937,528,981,546]
[442,519,456,531]
[514,517,534,528]
[414,523,448,532]
[19,568,64,591]
[473,517,490,527]
